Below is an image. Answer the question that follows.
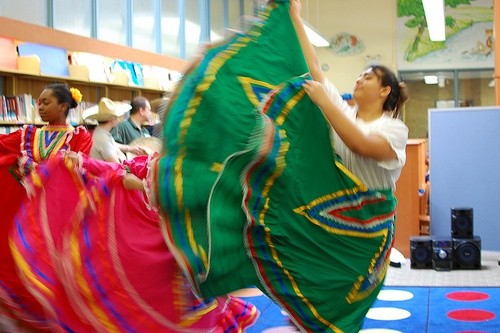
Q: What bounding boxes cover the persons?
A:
[147,0,409,333]
[0,84,262,333]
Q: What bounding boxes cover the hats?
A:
[126,136,163,161]
[81,97,133,121]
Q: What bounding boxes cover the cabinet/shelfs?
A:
[0,37,166,126]
[393,138,428,258]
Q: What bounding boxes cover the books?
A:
[0,92,99,137]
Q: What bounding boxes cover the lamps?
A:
[421,0,446,41]
[300,0,330,46]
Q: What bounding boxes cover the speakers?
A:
[451,207,474,239]
[410,237,432,270]
[432,236,454,271]
[452,236,481,270]
